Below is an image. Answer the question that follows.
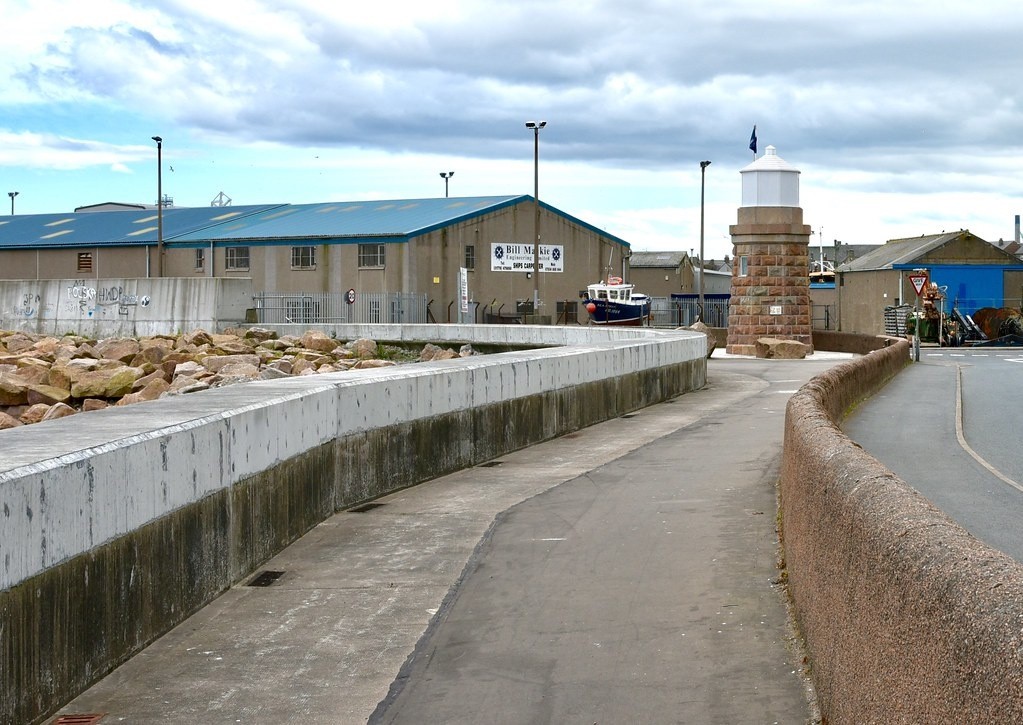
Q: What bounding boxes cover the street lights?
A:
[701,161,711,322]
[8,192,19,215]
[440,172,455,198]
[525,121,547,316]
[152,136,162,278]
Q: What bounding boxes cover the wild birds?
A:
[315,156,318,158]
[170,166,174,172]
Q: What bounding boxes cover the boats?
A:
[582,246,653,327]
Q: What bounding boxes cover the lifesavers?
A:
[607,275,623,285]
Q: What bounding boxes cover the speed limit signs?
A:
[348,289,356,303]
